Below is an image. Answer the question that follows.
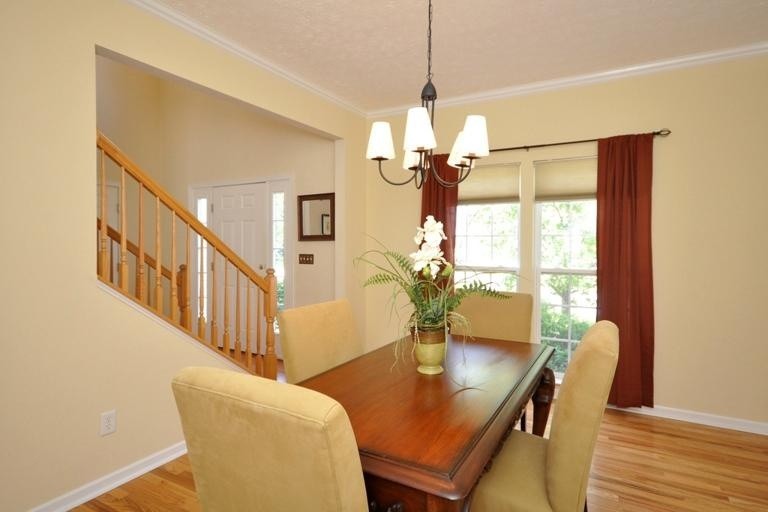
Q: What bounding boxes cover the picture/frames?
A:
[321,213,331,235]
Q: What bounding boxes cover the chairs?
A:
[450,287,534,432]
[171,365,370,511]
[278,299,362,384]
[468,319,619,510]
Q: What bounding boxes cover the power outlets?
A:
[100,408,117,437]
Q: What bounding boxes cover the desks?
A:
[292,333,556,510]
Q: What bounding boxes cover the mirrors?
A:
[297,193,334,241]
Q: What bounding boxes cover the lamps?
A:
[365,0,490,189]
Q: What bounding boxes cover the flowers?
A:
[356,213,514,332]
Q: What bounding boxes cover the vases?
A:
[408,323,450,375]
